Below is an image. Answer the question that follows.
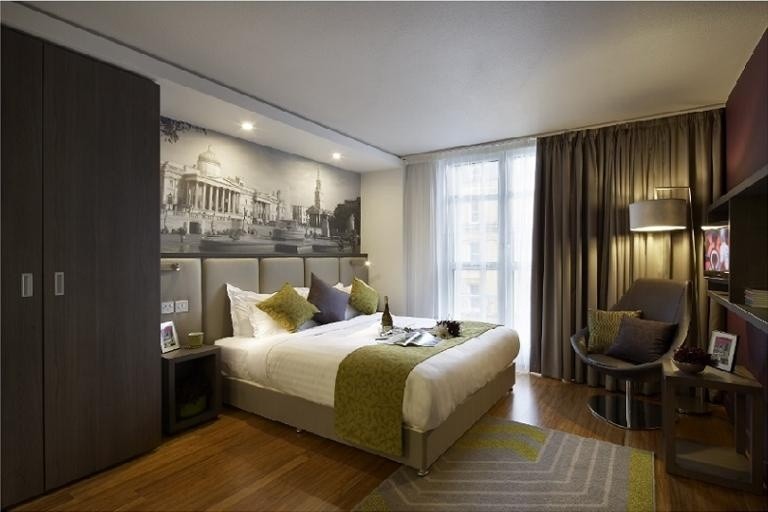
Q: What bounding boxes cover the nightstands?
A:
[164,344,222,435]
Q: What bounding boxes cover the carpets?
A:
[343,414,658,511]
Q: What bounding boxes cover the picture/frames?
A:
[160,321,180,352]
[708,329,740,372]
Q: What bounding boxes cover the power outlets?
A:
[161,301,174,313]
[174,300,188,313]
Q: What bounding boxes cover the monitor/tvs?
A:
[704,220,728,284]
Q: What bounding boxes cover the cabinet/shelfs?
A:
[0,22,163,510]
[702,162,767,493]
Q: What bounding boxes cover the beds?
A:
[217,311,521,479]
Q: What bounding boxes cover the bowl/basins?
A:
[671,359,706,374]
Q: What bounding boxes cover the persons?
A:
[704,227,729,273]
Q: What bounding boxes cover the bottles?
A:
[382,295,394,334]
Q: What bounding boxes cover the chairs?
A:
[568,274,694,430]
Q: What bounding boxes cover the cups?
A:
[187,332,204,348]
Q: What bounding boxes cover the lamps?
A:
[628,186,715,365]
[161,262,184,272]
[350,260,372,266]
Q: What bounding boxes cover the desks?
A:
[654,351,766,496]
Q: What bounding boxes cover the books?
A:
[382,331,442,347]
[744,286,768,308]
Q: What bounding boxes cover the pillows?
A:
[610,314,680,367]
[348,277,381,315]
[295,280,344,299]
[304,271,351,325]
[224,281,267,337]
[242,292,288,337]
[586,308,642,354]
[255,279,323,334]
[338,283,360,321]
